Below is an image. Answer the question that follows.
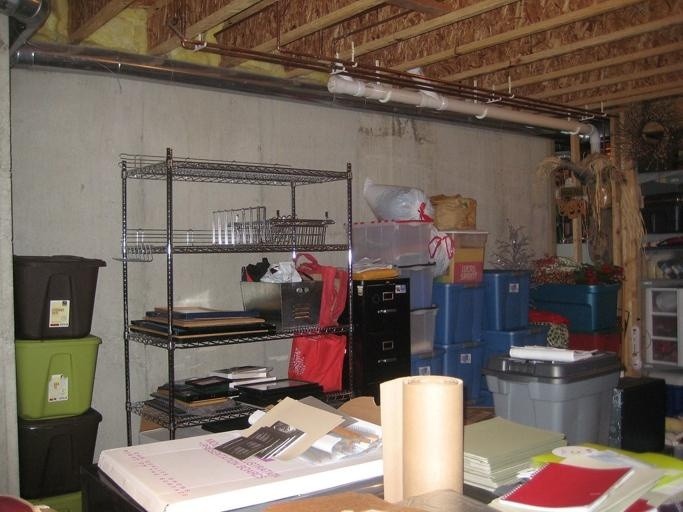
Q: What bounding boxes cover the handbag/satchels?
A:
[287,335,346,391]
[294,254,348,326]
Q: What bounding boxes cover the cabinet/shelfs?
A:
[645,286,681,368]
[113,148,353,446]
[642,205,683,233]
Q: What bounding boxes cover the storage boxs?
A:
[239,280,323,335]
[11,256,104,512]
[482,350,621,448]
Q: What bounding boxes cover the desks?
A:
[87,401,682,512]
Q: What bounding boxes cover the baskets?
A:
[227,219,335,245]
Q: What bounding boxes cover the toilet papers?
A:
[378,376,465,505]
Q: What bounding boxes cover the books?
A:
[129,303,277,344]
[213,419,308,461]
[143,365,280,418]
[507,344,599,365]
[462,415,682,511]
